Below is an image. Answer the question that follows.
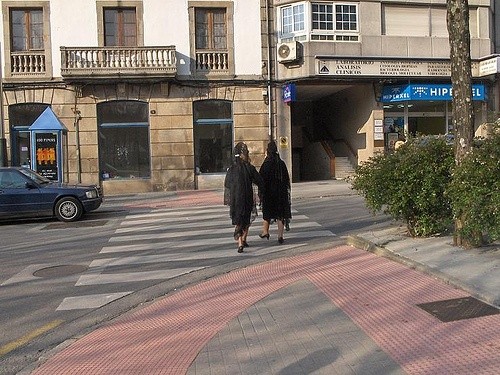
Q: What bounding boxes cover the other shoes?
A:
[238,246,243,253]
[242,241,248,248]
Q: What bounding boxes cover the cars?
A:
[0,166,105,222]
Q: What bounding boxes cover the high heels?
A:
[278,236,284,244]
[258,233,269,241]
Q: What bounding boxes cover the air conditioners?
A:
[277,41,303,62]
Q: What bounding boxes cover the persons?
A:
[258,141,292,243]
[224,142,263,253]
